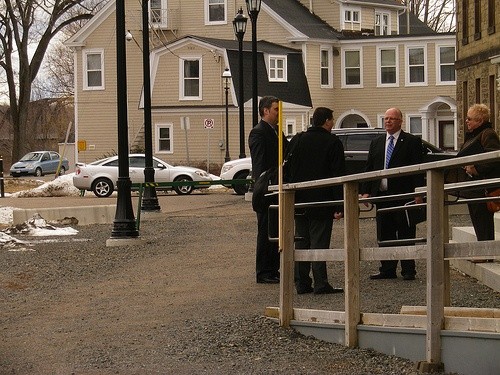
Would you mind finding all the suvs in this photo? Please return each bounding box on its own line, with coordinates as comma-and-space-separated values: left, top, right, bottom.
330, 128, 459, 196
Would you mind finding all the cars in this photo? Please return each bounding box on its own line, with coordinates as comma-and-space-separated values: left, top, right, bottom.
72, 154, 212, 198
10, 151, 69, 178
220, 134, 295, 195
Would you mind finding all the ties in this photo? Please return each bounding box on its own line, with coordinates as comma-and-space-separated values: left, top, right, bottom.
386, 136, 395, 168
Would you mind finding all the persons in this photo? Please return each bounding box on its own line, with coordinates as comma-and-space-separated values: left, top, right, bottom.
362, 108, 423, 280
248, 97, 290, 283
288, 107, 344, 295
455, 104, 500, 262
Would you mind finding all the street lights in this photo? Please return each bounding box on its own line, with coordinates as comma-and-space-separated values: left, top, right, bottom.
245, 0, 262, 201
232, 5, 248, 160
221, 67, 232, 163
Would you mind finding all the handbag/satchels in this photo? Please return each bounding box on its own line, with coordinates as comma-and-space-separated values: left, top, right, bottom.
484, 188, 500, 212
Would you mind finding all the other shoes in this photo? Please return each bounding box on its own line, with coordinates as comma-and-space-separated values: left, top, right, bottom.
403, 273, 415, 280
297, 286, 313, 294
257, 274, 281, 284
322, 286, 343, 293
467, 259, 493, 263
369, 271, 397, 278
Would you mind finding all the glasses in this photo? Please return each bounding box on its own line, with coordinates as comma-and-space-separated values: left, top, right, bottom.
465, 116, 472, 121
